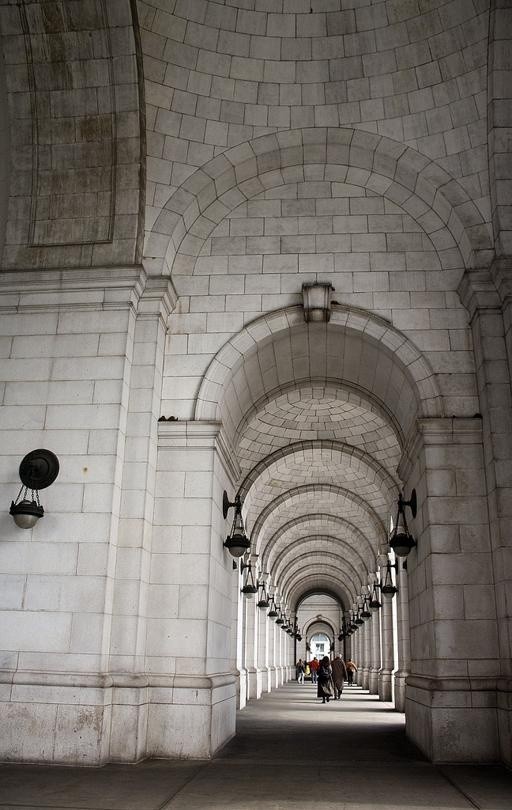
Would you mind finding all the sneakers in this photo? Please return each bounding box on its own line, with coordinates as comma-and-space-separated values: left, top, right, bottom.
298, 682, 305, 684
322, 697, 340, 703
348, 683, 353, 686
311, 681, 317, 684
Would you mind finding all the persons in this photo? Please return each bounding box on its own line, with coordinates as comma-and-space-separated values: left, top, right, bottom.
296, 656, 358, 703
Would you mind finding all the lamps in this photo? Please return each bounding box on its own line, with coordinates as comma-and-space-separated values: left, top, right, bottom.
298, 280, 334, 322
353, 489, 417, 626
9, 448, 59, 529
220, 489, 285, 627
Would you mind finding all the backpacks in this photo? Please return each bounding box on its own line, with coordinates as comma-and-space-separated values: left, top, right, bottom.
321, 666, 331, 681
